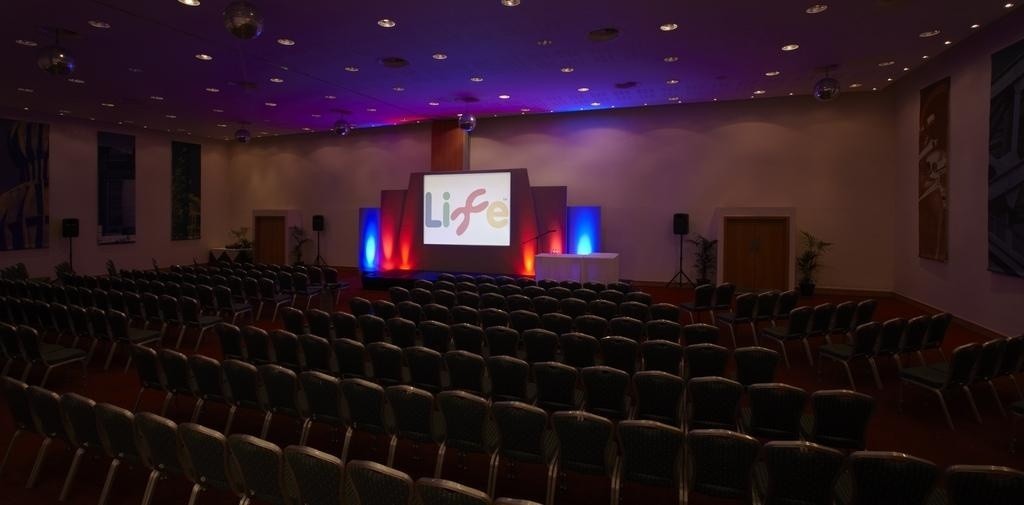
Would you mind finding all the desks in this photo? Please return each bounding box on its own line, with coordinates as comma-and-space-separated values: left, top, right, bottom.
535, 253, 620, 284
210, 248, 253, 264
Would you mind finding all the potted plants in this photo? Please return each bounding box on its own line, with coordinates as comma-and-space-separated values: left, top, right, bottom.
688, 232, 718, 286
795, 232, 833, 295
289, 225, 306, 266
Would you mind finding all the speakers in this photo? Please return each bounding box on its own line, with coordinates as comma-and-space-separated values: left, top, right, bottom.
312, 214, 324, 232
62, 218, 79, 238
672, 212, 690, 235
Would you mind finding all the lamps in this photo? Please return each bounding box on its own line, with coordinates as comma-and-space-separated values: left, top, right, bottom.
234, 121, 252, 143
812, 65, 841, 102
454, 96, 480, 133
333, 109, 351, 137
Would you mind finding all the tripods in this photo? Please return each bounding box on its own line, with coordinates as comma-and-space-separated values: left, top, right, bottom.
314, 236, 327, 266
664, 241, 695, 288
